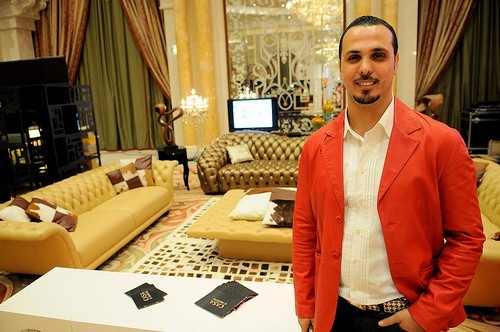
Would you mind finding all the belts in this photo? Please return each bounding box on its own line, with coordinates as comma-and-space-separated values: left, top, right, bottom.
346, 297, 410, 314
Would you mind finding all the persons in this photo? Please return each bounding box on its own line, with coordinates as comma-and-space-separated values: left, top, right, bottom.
291, 16, 486, 332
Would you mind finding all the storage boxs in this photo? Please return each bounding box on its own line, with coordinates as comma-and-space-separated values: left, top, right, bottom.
460, 111, 500, 154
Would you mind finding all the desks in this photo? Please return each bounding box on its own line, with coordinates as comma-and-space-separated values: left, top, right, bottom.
0, 266, 302, 332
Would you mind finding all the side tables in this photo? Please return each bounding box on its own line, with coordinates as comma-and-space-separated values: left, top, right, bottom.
158, 146, 190, 191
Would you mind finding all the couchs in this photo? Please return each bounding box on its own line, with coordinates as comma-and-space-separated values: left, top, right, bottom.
0, 159, 178, 275
197, 132, 309, 194
462, 154, 500, 307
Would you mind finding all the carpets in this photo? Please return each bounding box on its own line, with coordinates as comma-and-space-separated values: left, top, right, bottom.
129, 197, 292, 284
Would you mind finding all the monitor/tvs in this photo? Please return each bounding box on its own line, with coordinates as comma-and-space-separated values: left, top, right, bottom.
227, 98, 278, 132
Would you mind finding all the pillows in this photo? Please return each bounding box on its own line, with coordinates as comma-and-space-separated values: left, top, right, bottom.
105, 162, 143, 194
25, 198, 78, 232
225, 144, 253, 163
119, 154, 156, 186
0, 195, 36, 222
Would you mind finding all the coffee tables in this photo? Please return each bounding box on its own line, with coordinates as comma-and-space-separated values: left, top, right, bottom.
188, 189, 292, 263
